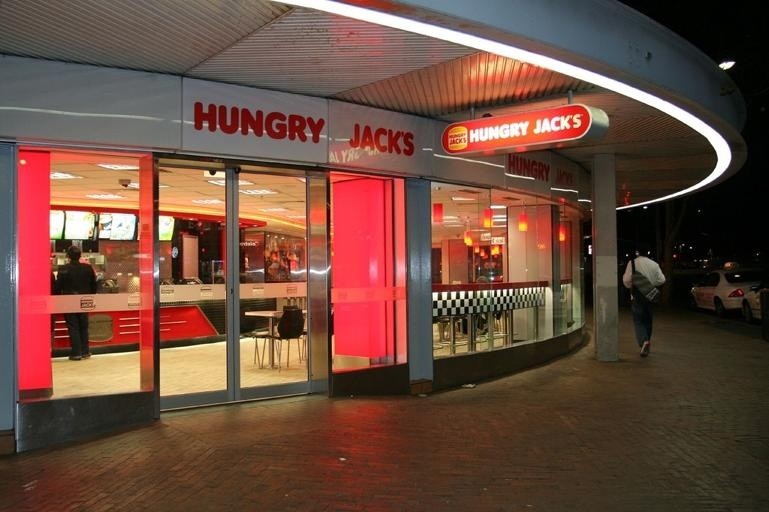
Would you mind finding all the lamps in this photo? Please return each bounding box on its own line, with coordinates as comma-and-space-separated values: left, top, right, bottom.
433, 187, 566, 261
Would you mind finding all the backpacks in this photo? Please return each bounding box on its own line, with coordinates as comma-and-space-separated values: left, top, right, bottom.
631, 259, 661, 308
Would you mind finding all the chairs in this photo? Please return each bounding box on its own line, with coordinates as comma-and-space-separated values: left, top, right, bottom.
433, 311, 516, 356
252, 302, 334, 373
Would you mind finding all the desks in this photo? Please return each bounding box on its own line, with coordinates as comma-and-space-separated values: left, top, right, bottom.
245, 310, 307, 370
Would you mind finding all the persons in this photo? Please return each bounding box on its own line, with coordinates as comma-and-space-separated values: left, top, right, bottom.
264, 251, 290, 283
620, 241, 667, 360
56, 245, 99, 360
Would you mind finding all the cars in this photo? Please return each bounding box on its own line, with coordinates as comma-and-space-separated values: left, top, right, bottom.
689, 262, 769, 322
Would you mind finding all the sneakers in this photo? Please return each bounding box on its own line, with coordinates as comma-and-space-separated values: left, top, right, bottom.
69, 350, 92, 361
639, 340, 652, 357
